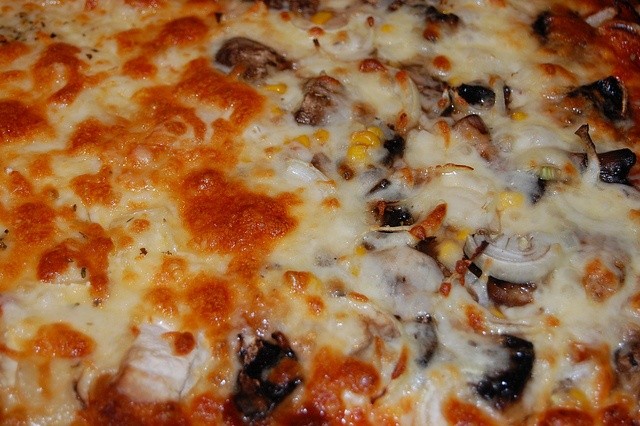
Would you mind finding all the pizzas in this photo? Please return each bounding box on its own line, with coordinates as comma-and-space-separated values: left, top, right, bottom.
0, 0, 636, 426
